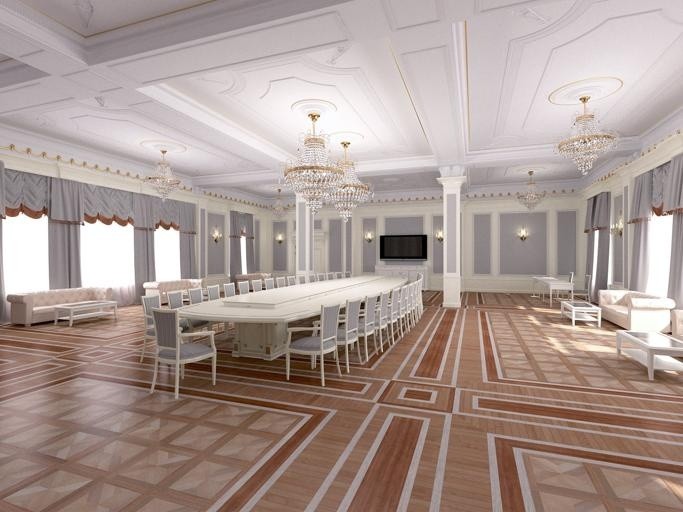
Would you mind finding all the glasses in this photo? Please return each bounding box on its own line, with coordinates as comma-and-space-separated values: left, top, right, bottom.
283, 99, 346, 216
364, 229, 375, 243
211, 224, 223, 244
144, 150, 181, 202
436, 229, 443, 243
515, 171, 549, 210
615, 215, 624, 237
329, 132, 374, 223
516, 225, 528, 241
275, 232, 284, 244
557, 96, 618, 176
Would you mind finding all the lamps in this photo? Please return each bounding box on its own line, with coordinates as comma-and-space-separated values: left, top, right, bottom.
670, 309, 683, 342
556, 272, 574, 302
568, 275, 591, 303
150, 306, 217, 400
140, 271, 423, 386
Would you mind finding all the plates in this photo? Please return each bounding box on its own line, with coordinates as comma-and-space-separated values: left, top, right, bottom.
375, 265, 427, 292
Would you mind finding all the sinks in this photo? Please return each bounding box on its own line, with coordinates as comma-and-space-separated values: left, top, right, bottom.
598, 290, 676, 329
7, 288, 113, 327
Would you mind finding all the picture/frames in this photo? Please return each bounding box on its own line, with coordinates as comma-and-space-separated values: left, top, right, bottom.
528, 275, 573, 308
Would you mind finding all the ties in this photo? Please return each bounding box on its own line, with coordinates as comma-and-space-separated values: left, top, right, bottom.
380, 235, 428, 260
